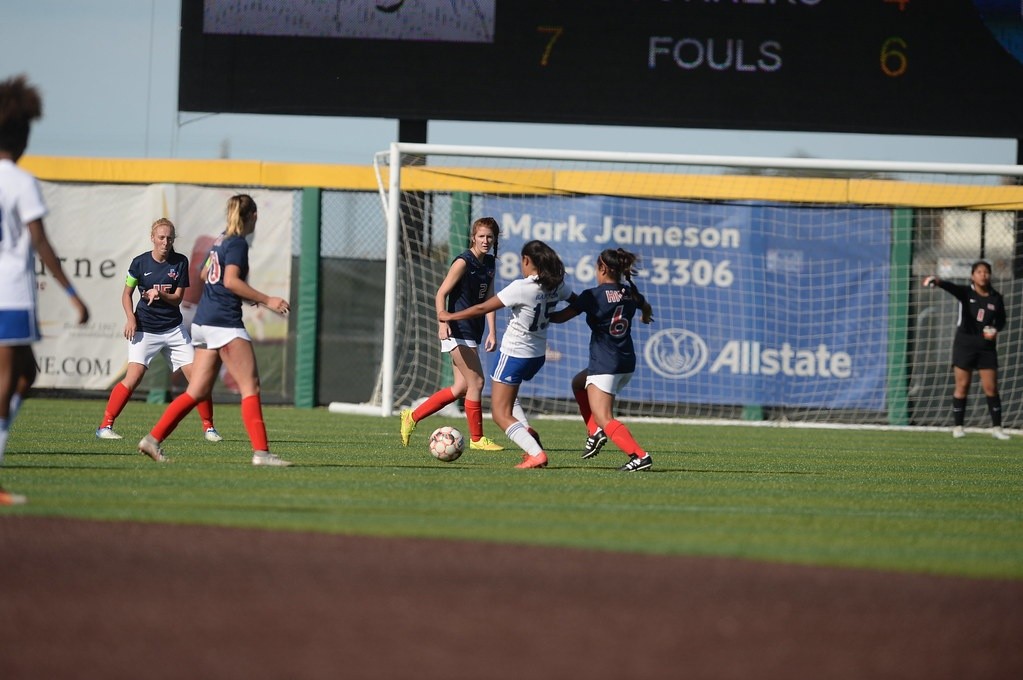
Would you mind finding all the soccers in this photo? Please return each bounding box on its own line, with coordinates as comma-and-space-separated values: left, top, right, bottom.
429, 426, 465, 462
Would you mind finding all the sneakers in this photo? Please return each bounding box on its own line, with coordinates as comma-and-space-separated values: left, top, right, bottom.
205, 427, 223, 442
526, 426, 544, 449
138, 435, 170, 463
515, 450, 548, 468
620, 451, 652, 473
470, 435, 505, 450
581, 429, 608, 459
399, 409, 418, 447
252, 452, 294, 467
95, 426, 124, 440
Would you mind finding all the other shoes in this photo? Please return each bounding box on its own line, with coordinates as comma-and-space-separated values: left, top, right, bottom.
952, 425, 965, 439
990, 427, 1011, 440
0, 485, 26, 504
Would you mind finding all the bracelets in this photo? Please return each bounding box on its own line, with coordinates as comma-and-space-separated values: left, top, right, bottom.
66, 286, 75, 297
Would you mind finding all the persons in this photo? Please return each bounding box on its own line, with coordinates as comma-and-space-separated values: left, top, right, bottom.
400, 217, 504, 451
0, 72, 90, 504
550, 248, 655, 472
922, 261, 1010, 440
137, 194, 292, 467
95, 218, 223, 442
438, 241, 579, 469
